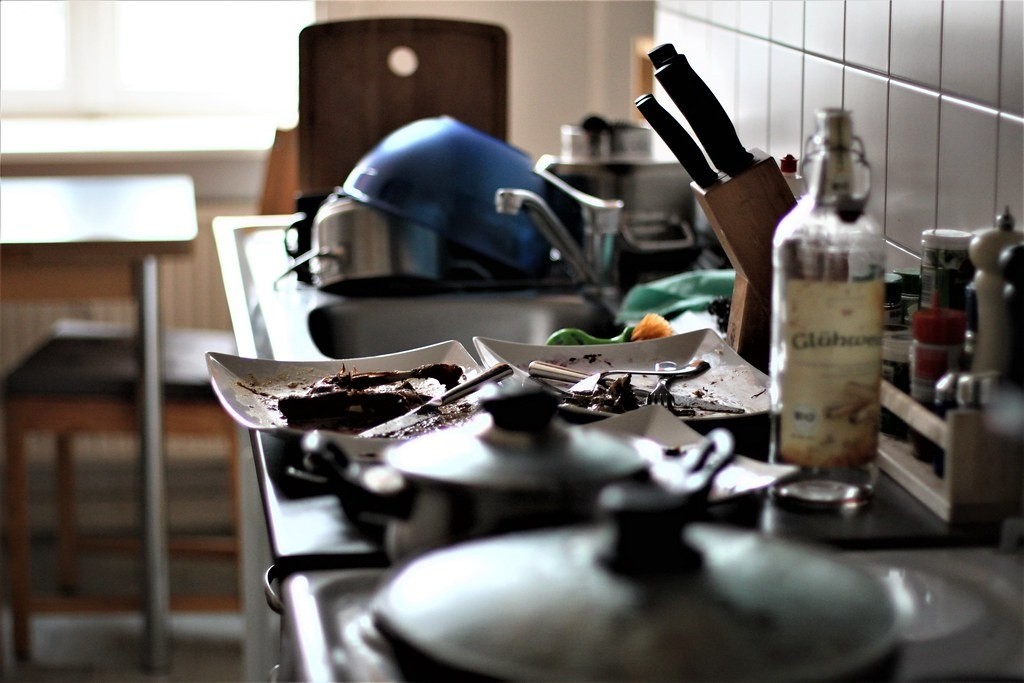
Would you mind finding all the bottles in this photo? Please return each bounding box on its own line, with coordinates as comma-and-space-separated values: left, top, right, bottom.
882, 268, 922, 323
770, 108, 886, 509
775, 155, 803, 204
910, 310, 968, 469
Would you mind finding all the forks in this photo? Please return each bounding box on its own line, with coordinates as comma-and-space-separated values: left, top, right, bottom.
647, 361, 677, 411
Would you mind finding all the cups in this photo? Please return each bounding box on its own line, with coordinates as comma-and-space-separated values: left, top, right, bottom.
284, 193, 328, 284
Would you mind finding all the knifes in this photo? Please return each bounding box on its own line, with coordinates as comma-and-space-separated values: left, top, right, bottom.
355, 363, 514, 437
528, 360, 745, 413
634, 42, 753, 184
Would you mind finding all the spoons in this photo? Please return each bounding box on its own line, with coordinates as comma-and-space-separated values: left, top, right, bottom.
569, 359, 711, 391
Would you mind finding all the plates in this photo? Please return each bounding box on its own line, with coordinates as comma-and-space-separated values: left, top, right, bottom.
472, 328, 770, 420
205, 340, 502, 440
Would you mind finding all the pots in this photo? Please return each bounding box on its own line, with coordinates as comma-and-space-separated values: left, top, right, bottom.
301, 385, 736, 568
370, 484, 901, 683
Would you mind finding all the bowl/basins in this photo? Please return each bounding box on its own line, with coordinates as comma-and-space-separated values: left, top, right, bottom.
310, 114, 551, 293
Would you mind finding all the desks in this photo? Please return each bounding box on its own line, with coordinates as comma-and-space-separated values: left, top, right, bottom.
0, 171, 200, 673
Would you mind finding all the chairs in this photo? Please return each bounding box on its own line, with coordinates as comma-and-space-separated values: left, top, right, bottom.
0, 117, 301, 664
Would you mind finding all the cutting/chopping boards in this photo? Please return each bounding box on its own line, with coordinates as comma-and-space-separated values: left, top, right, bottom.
297, 17, 509, 187
